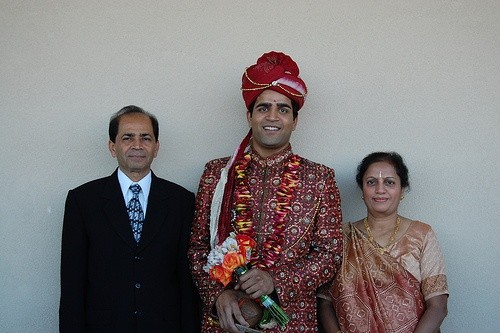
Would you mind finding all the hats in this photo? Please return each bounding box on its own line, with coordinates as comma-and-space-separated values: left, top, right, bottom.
242, 51, 307, 110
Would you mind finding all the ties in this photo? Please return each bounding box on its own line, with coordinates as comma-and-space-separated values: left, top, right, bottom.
127, 184, 145, 246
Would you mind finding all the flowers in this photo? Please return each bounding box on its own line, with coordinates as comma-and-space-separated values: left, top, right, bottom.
203, 230, 292, 330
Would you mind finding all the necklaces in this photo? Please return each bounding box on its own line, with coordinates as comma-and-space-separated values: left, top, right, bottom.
362, 215, 402, 255
232, 150, 302, 267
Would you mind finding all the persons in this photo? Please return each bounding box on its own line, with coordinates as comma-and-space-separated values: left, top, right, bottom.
58, 104, 206, 332
187, 50, 343, 333
317, 152, 449, 333
